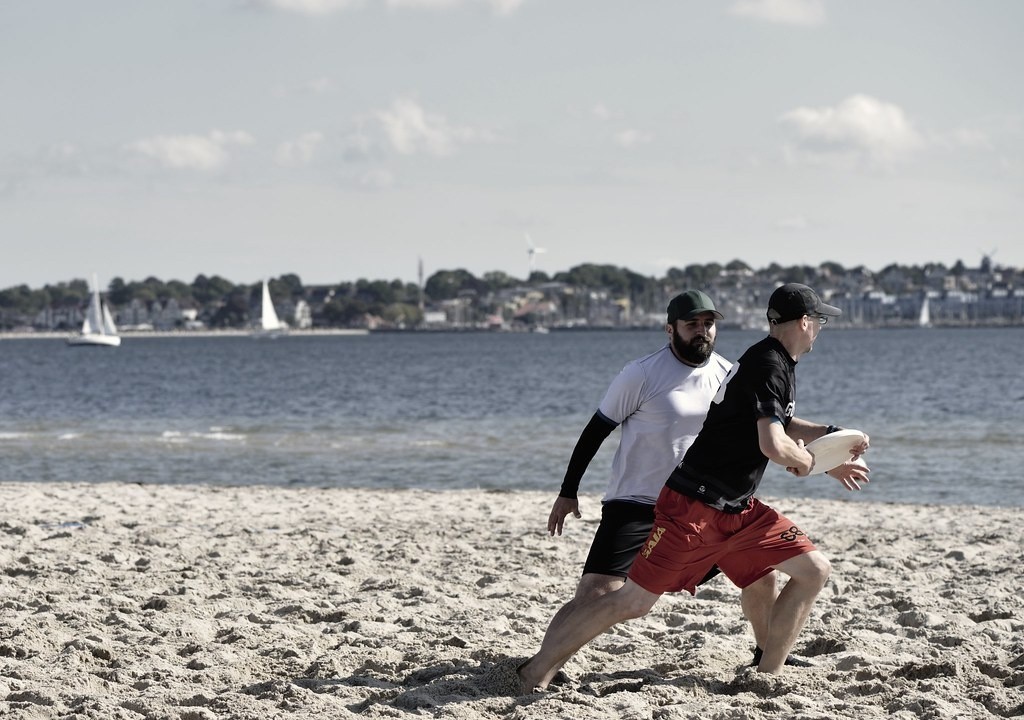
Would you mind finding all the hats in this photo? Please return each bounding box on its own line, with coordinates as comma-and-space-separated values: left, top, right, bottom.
767, 283, 842, 325
667, 290, 724, 320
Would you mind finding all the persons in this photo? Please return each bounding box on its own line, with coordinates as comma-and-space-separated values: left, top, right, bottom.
516, 283, 869, 695
543, 291, 871, 670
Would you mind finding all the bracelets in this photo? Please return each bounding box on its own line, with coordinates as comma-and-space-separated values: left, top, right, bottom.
827, 425, 840, 436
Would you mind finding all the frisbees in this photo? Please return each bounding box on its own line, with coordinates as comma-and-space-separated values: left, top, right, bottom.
805, 429, 865, 476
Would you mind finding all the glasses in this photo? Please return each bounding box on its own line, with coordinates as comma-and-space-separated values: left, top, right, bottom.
810, 315, 828, 324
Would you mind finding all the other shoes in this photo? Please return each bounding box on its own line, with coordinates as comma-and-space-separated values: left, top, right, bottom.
517, 661, 576, 685
751, 646, 815, 668
